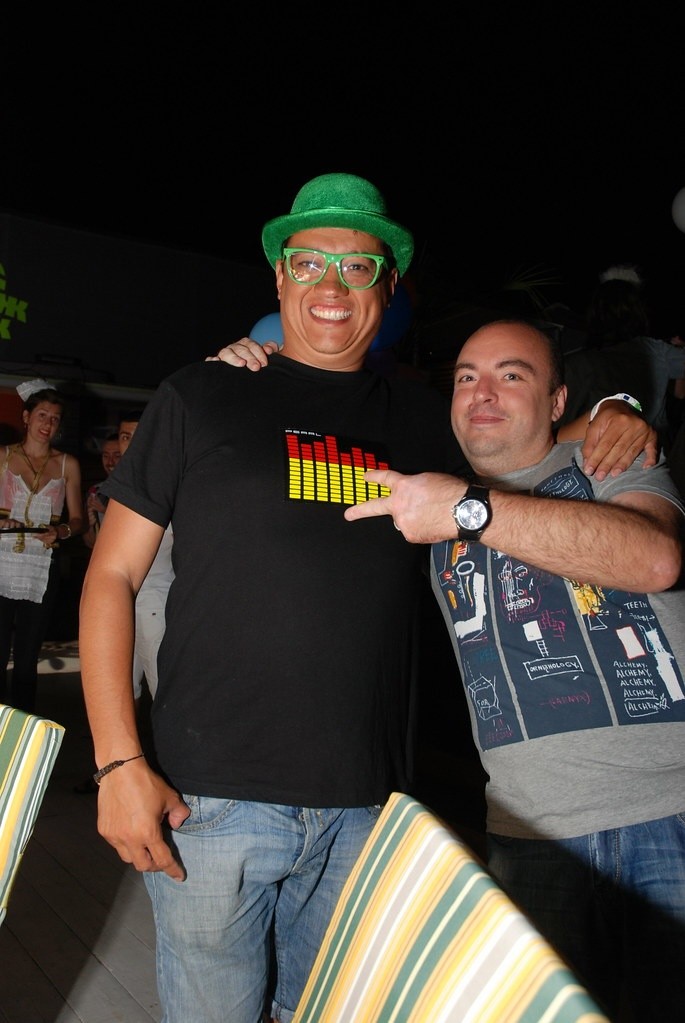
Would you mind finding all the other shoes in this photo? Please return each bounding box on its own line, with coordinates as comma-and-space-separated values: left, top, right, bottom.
74, 778, 99, 793
80, 722, 92, 737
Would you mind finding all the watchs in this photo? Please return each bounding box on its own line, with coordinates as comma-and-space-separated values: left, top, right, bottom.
452, 483, 492, 541
590, 393, 642, 422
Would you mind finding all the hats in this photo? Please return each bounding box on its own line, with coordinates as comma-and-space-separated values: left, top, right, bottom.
262, 173, 414, 279
16, 379, 56, 403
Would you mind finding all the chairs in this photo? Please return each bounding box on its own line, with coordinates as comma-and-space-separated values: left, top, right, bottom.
0, 703, 65, 926
288, 791, 617, 1023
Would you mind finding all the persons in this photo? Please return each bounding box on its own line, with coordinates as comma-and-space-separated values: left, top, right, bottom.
204, 318, 685, 1023
568, 280, 684, 458
79, 174, 684, 1022
0, 379, 145, 713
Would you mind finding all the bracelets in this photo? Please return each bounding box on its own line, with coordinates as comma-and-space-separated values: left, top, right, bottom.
93, 754, 144, 785
60, 524, 72, 539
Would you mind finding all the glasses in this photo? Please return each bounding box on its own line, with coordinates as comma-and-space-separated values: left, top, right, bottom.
282, 248, 389, 290
